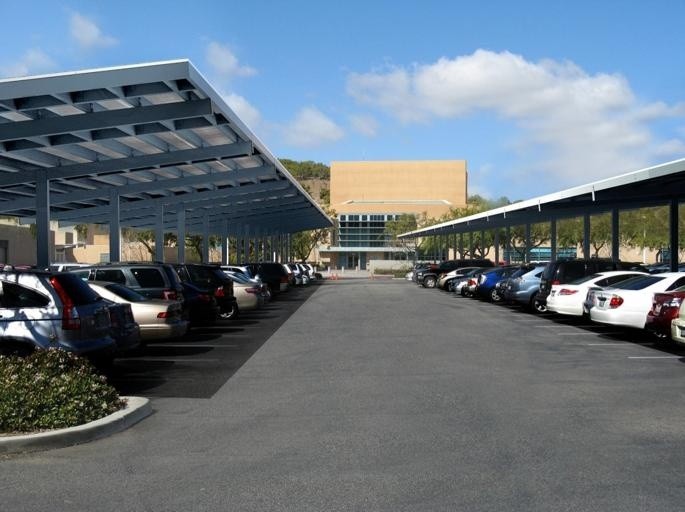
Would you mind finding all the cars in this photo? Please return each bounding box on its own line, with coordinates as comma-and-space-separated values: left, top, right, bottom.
410, 256, 685, 346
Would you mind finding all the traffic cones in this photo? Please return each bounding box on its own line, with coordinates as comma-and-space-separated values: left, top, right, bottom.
331, 273, 334, 281
335, 273, 339, 280
